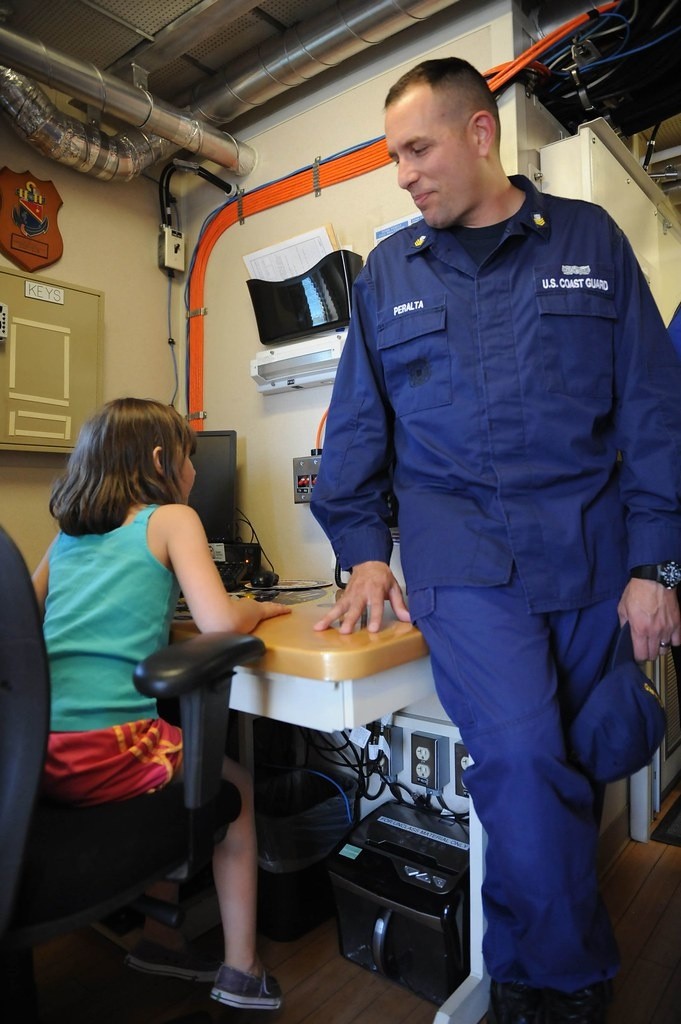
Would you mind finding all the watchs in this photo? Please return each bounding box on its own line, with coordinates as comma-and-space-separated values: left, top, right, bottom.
630, 560, 681, 590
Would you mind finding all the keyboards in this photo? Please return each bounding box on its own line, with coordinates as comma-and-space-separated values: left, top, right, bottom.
215, 560, 248, 592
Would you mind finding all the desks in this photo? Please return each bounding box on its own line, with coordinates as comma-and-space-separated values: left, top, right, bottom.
174, 577, 436, 734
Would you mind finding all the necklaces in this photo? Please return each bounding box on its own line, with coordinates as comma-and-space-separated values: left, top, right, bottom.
660, 641, 672, 648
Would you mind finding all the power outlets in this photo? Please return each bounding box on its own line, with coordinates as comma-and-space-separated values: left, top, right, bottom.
411, 731, 450, 790
454, 740, 470, 798
365, 725, 403, 776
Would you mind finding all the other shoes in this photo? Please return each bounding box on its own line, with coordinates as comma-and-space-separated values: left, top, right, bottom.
478, 982, 612, 1024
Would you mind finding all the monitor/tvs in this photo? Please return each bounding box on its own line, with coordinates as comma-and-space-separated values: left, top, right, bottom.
185, 430, 236, 543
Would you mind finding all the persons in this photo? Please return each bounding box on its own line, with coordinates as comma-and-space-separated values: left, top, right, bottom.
31, 398, 291, 1009
309, 56, 681, 1024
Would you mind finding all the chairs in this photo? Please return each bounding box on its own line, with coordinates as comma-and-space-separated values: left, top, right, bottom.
0, 520, 267, 1024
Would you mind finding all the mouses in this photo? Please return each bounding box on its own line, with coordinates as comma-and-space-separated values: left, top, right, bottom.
251, 571, 279, 589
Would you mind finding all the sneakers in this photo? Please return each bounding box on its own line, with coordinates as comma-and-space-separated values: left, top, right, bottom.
210, 963, 283, 1010
124, 938, 218, 982
